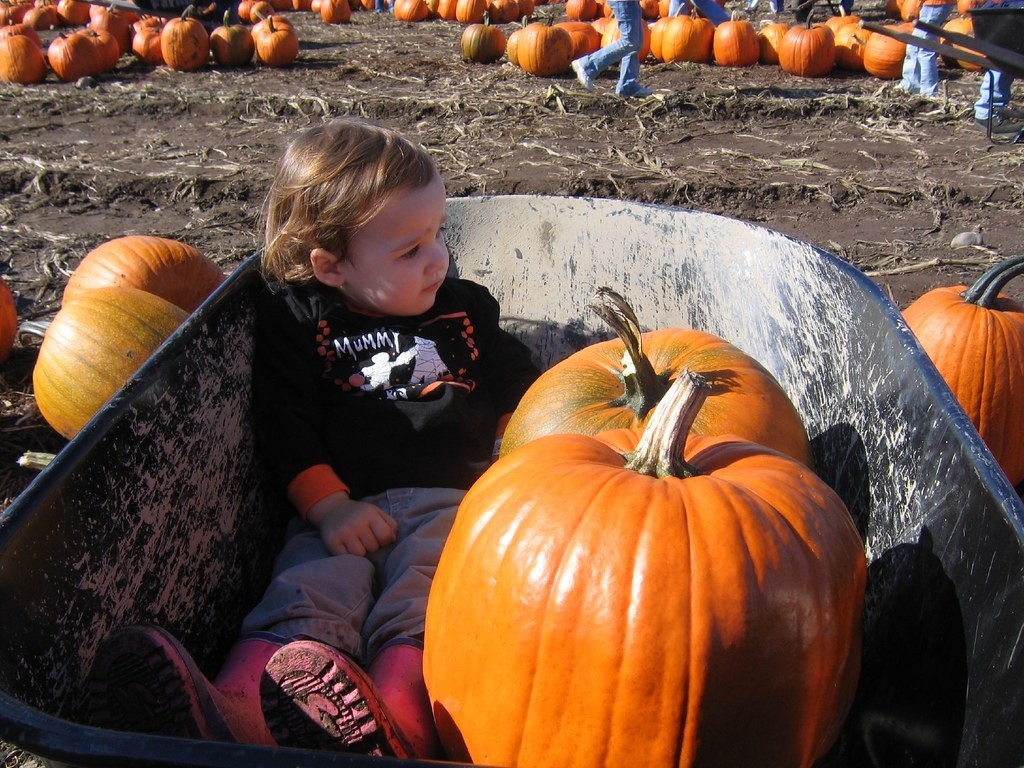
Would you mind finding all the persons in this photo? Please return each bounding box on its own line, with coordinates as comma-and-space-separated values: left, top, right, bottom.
97, 121, 541, 758
669, 0, 730, 27
895, 0, 959, 100
373, 0, 395, 14
747, 0, 785, 17
838, 0, 854, 17
972, 0, 1024, 139
181, 0, 241, 25
572, 0, 654, 98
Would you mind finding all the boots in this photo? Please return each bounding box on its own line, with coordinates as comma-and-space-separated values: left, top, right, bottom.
88, 623, 284, 749
260, 638, 443, 760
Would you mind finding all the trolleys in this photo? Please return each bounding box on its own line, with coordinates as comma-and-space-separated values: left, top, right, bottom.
0, 191, 1024, 768
858, 7, 1024, 146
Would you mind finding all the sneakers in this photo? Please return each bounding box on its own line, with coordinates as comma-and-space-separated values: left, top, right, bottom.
633, 88, 652, 97
995, 106, 1024, 120
976, 112, 1023, 138
571, 60, 594, 92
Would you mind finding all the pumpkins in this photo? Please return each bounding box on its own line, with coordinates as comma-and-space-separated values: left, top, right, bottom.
899, 254, 1024, 491
0, 0, 998, 86
423, 367, 869, 768
0, 234, 233, 439
499, 288, 812, 472
